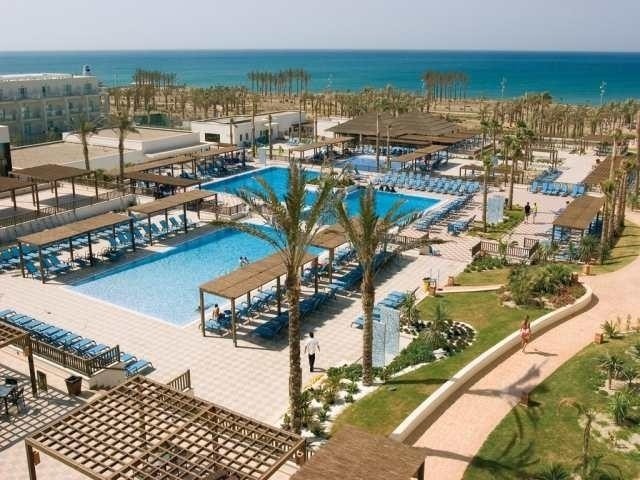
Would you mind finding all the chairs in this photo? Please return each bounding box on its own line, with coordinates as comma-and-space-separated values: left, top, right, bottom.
311, 142, 479, 235
526, 178, 604, 243
1, 147, 246, 282
0, 308, 155, 417
202, 246, 410, 346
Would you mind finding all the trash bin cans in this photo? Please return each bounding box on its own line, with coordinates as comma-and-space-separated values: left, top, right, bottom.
65, 376, 83, 396
571, 272, 578, 282
595, 334, 603, 344
448, 276, 454, 286
583, 265, 590, 275
